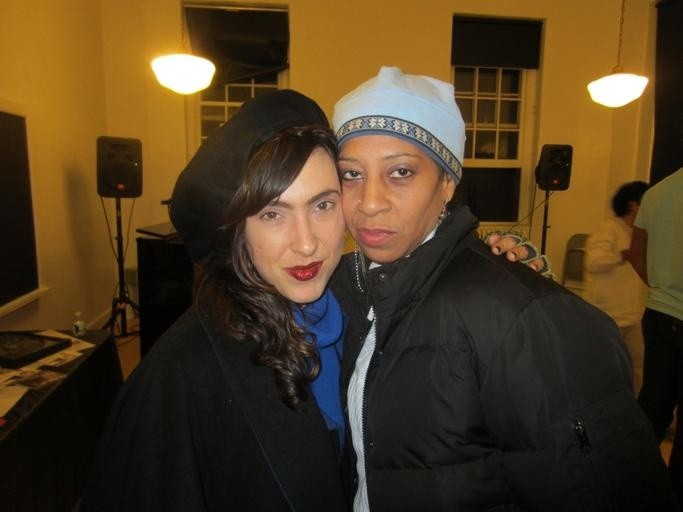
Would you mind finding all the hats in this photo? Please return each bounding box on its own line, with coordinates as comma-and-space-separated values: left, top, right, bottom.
169, 90, 330, 263
332, 66, 467, 182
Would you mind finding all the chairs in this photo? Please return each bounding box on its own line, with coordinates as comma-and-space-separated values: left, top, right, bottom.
558, 233, 590, 291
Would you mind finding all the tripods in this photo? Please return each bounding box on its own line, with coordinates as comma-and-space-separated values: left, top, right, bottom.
101, 198, 140, 336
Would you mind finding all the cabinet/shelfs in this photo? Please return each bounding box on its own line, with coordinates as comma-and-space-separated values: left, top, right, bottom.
0, 327, 127, 511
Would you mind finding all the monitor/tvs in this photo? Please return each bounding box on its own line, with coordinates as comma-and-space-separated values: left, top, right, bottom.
1, 111, 40, 307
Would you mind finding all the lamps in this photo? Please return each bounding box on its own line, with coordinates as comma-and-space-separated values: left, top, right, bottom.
149, 1, 217, 97
585, 1, 651, 112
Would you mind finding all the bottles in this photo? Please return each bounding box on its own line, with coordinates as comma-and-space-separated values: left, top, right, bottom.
72, 311, 87, 338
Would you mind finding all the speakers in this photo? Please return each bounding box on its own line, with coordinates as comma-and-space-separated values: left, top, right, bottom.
97, 136, 143, 198
534, 144, 572, 190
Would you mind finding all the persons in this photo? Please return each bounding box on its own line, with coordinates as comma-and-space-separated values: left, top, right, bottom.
95, 86, 558, 512
626, 160, 682, 490
578, 182, 646, 402
329, 65, 682, 512
